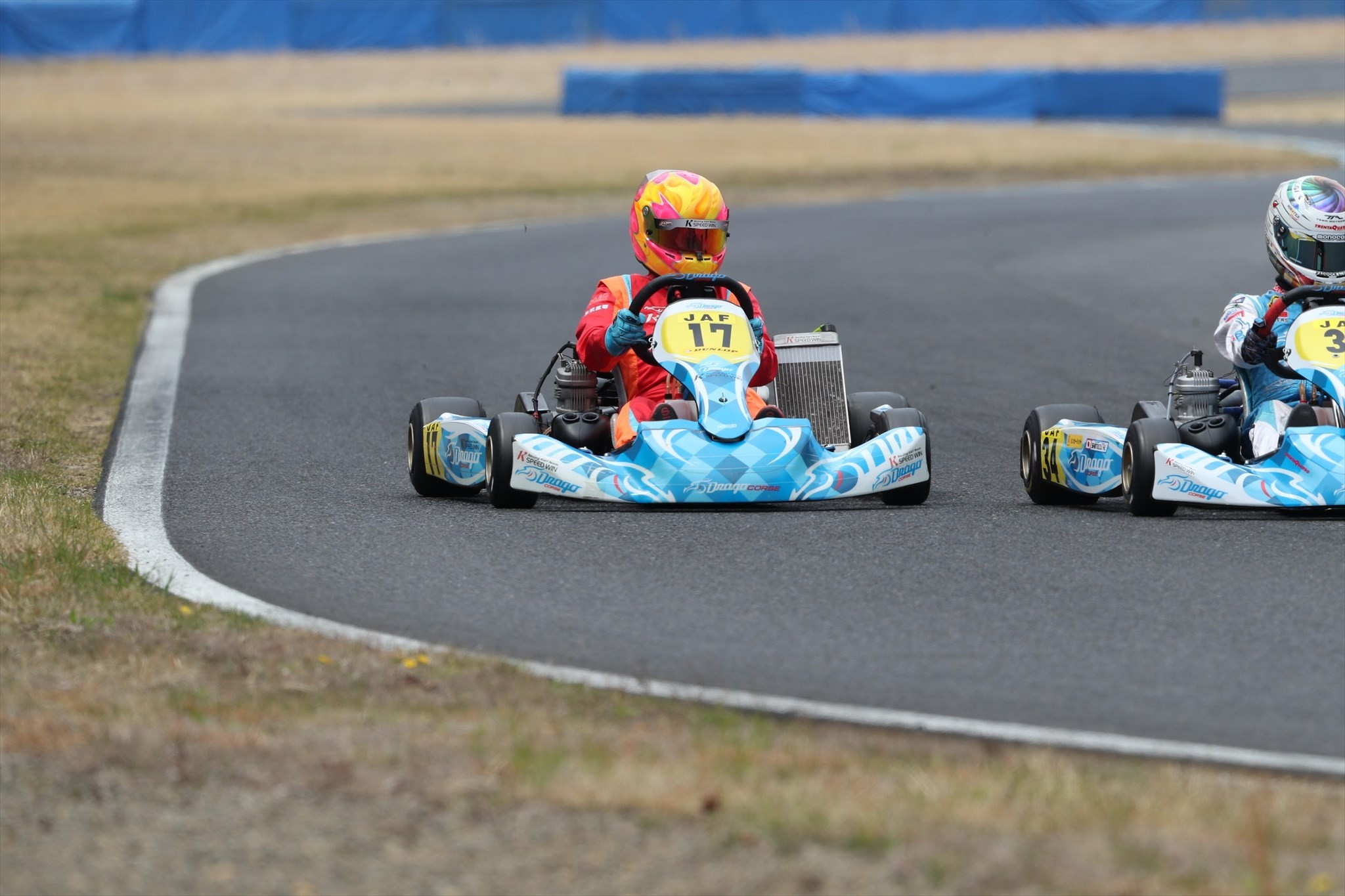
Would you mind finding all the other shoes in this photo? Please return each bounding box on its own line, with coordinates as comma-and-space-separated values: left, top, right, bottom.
1283, 402, 1318, 431
649, 403, 679, 421
754, 404, 785, 420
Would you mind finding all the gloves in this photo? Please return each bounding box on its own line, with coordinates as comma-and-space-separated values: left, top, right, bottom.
750, 317, 764, 354
1242, 318, 1277, 365
604, 308, 648, 356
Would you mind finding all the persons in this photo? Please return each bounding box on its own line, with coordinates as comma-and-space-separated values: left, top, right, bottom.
1214, 176, 1345, 465
575, 170, 785, 452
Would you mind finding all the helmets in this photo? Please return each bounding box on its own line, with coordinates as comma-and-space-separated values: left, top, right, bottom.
629, 170, 728, 276
1264, 175, 1345, 291
